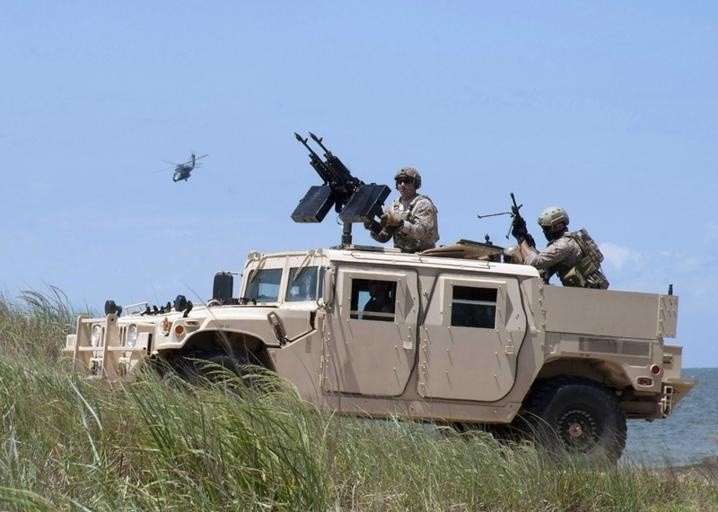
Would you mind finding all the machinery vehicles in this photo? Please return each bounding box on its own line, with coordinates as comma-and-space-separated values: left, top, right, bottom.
50, 128, 700, 473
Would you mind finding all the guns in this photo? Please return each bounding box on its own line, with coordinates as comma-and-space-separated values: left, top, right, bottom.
295, 132, 396, 242
477, 192, 549, 284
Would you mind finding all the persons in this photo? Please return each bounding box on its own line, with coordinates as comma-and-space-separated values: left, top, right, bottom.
365, 167, 439, 254
510, 206, 608, 289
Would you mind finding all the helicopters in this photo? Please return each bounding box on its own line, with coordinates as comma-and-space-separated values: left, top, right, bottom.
150, 151, 209, 185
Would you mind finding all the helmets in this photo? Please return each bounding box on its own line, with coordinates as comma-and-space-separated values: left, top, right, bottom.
536, 206, 570, 227
503, 246, 522, 263
394, 167, 422, 188
